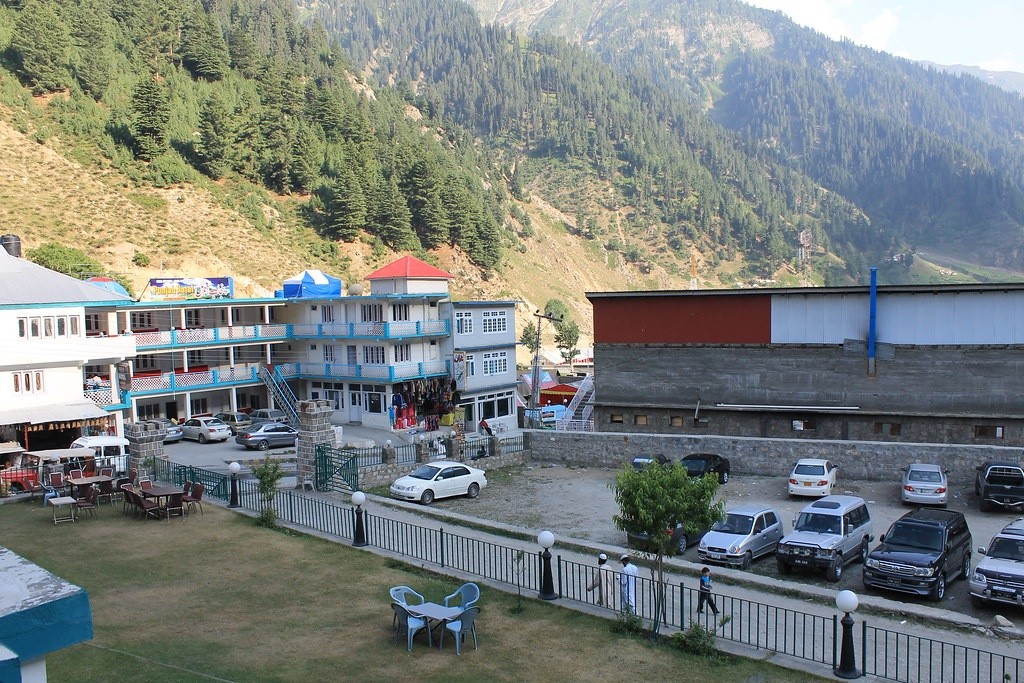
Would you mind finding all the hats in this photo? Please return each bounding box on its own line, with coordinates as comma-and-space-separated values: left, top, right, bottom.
621, 555, 629, 561
599, 554, 607, 559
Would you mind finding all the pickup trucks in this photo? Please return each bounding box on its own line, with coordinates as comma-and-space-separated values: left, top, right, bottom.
974, 459, 1024, 514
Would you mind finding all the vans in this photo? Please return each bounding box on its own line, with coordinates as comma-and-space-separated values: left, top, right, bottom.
70, 435, 130, 478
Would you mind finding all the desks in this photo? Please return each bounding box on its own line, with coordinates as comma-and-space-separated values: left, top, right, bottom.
409, 602, 458, 646
48, 496, 76, 524
68, 475, 111, 504
139, 487, 185, 518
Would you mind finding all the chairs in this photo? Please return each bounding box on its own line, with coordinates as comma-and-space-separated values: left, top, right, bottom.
114, 468, 204, 518
24, 468, 113, 504
390, 585, 432, 651
440, 583, 481, 655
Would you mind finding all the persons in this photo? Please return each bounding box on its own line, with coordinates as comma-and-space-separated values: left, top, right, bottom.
478, 446, 485, 454
436, 437, 446, 454
696, 567, 720, 615
588, 554, 613, 609
480, 417, 493, 436
88, 374, 101, 382
617, 555, 638, 615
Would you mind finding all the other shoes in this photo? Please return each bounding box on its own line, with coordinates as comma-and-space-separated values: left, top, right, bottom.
714, 611, 719, 613
697, 609, 703, 613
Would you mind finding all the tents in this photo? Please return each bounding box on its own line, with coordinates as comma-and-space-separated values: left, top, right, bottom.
283, 270, 341, 298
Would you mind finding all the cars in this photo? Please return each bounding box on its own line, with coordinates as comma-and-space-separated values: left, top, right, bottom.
389, 460, 489, 506
899, 463, 951, 508
235, 423, 302, 451
626, 518, 720, 555
696, 505, 784, 570
207, 410, 252, 436
629, 453, 672, 474
178, 416, 233, 444
786, 458, 838, 499
136, 418, 183, 442
248, 408, 289, 425
673, 452, 731, 484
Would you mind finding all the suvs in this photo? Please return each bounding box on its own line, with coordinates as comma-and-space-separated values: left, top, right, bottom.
0, 448, 96, 496
774, 494, 874, 584
967, 516, 1024, 609
861, 506, 974, 603
